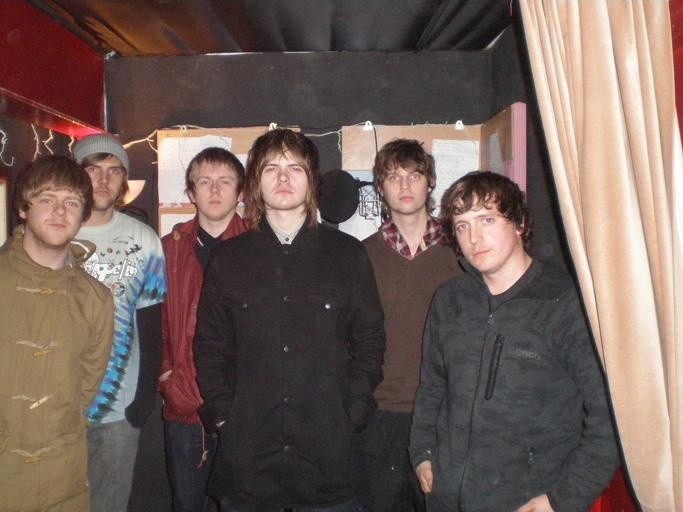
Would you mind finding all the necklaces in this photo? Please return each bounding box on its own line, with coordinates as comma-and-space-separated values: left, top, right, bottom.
270, 223, 304, 242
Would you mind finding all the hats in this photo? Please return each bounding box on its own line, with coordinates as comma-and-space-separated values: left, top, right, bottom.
73, 134, 130, 170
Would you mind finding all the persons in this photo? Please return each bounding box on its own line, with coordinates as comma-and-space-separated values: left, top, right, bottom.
357, 138, 465, 511
64, 133, 168, 510
192, 127, 385, 512
0, 154, 114, 511
158, 147, 256, 509
408, 169, 624, 512
